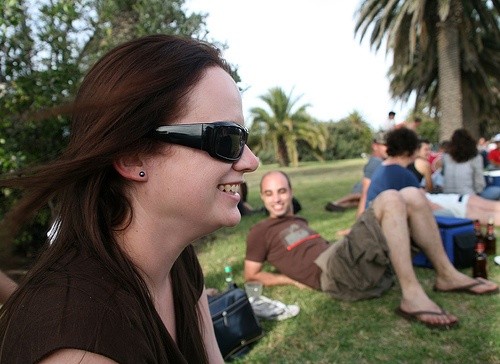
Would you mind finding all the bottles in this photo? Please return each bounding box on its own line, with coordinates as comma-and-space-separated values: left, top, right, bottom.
222, 266, 235, 294
473, 216, 496, 280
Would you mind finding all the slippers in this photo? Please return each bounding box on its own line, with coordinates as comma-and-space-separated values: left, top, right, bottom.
433, 279, 500, 295
394, 302, 458, 328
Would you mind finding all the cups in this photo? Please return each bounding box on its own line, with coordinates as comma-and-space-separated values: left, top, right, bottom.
244, 281, 263, 298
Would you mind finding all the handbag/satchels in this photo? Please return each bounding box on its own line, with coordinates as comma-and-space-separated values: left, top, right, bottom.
207, 284, 265, 359
411, 215, 477, 269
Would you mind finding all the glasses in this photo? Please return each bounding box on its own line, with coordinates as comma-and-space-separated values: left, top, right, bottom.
147, 121, 249, 164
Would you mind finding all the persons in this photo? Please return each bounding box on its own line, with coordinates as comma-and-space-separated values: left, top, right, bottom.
357, 132, 499, 227
379, 111, 398, 134
334, 182, 363, 207
405, 140, 444, 194
0, 34, 258, 364
439, 129, 500, 201
365, 129, 455, 218
243, 170, 500, 329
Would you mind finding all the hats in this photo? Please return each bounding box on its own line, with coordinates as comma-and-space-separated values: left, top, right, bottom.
373, 131, 387, 144
